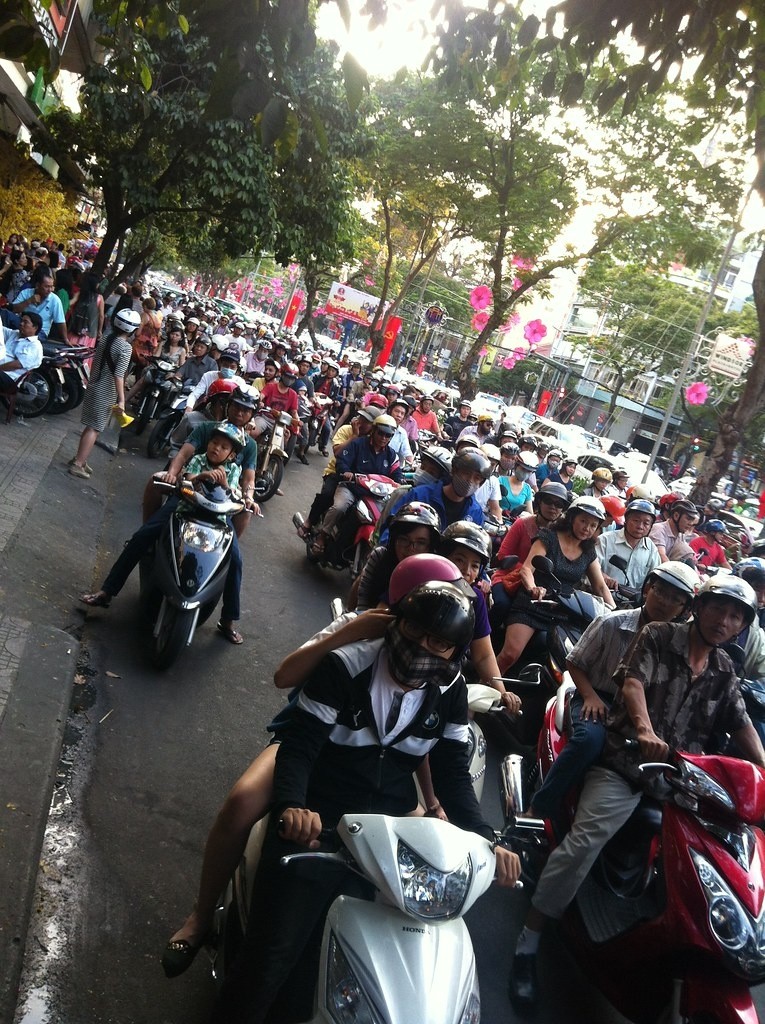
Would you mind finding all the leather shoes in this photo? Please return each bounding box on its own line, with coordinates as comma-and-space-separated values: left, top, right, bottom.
507, 952, 539, 1015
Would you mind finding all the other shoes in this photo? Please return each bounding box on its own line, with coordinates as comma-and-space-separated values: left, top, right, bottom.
160, 924, 216, 979
68, 455, 93, 480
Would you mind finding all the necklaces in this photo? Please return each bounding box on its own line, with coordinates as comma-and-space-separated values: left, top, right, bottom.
691, 654, 709, 679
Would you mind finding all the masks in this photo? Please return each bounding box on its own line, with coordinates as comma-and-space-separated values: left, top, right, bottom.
515, 467, 533, 482
451, 472, 480, 498
501, 459, 516, 470
282, 376, 295, 387
258, 350, 268, 361
412, 467, 440, 487
388, 627, 461, 689
220, 367, 236, 378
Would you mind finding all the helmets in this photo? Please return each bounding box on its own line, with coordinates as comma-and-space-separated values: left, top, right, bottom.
650, 560, 701, 600
438, 521, 494, 561
30, 241, 763, 600
390, 579, 476, 656
195, 334, 212, 353
387, 501, 442, 530
697, 574, 758, 624
387, 552, 478, 607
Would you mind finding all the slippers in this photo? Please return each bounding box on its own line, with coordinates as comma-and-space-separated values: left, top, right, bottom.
217, 621, 243, 644
78, 595, 110, 610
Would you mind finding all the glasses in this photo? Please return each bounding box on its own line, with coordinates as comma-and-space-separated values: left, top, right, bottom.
651, 583, 687, 607
541, 497, 565, 510
377, 430, 393, 438
399, 614, 458, 653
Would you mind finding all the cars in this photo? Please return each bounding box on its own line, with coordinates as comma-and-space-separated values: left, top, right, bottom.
157, 282, 189, 303
469, 391, 765, 557
213, 296, 461, 408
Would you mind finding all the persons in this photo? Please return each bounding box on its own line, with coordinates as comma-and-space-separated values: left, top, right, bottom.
0, 233, 765, 1009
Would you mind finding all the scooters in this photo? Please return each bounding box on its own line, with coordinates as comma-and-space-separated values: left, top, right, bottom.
0, 338, 99, 424
138, 473, 265, 673
130, 352, 343, 503
288, 407, 765, 749
201, 817, 548, 1024
499, 672, 765, 1024
